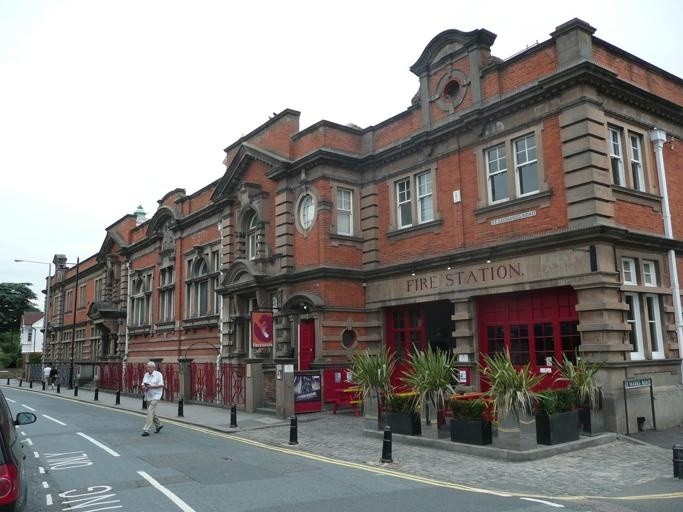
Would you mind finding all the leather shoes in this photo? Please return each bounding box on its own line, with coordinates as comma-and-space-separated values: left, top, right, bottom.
142, 432, 148, 436
154, 426, 162, 433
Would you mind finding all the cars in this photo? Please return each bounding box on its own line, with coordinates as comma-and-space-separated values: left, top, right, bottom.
0, 391, 37, 511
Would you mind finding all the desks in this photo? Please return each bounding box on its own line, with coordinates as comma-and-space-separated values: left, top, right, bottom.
332, 389, 363, 417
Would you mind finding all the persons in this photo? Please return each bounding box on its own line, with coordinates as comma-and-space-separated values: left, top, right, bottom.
139, 361, 164, 437
42, 364, 51, 391
49, 365, 57, 390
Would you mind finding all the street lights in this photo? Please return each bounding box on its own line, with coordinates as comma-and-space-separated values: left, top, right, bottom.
21, 326, 36, 352
15, 259, 50, 365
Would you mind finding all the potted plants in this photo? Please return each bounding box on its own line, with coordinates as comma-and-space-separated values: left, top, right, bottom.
341, 343, 610, 452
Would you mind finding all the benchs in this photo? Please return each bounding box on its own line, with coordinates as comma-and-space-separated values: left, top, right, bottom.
327, 398, 363, 417
